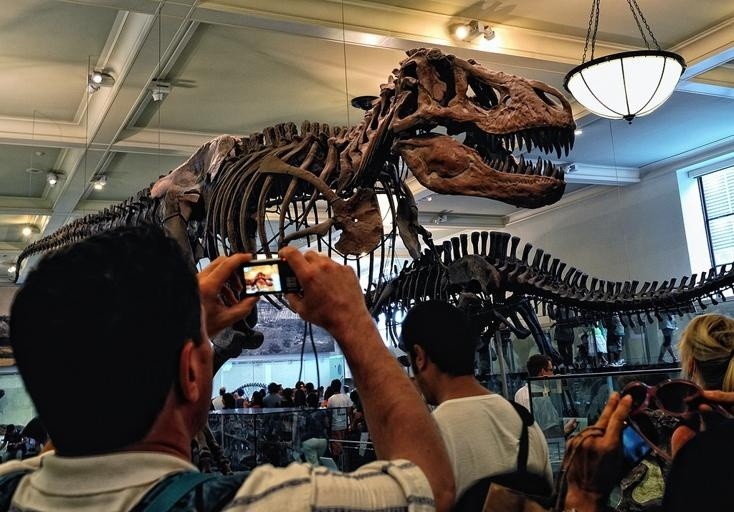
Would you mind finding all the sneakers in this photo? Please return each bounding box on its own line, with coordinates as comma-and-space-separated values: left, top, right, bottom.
609, 361, 623, 367
603, 361, 615, 368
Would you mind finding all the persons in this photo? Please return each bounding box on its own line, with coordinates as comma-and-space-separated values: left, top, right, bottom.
191, 379, 377, 475
0, 225, 456, 512
514, 312, 734, 512
0, 424, 27, 460
401, 301, 554, 512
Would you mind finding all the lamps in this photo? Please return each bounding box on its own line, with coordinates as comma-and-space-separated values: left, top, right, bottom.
86, 71, 116, 94
562, 1, 686, 126
149, 78, 170, 102
455, 19, 495, 44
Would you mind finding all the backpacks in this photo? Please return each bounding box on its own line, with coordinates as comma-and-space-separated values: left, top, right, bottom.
454, 471, 552, 512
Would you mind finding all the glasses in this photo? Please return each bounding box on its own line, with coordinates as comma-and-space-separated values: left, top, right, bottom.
620, 380, 734, 464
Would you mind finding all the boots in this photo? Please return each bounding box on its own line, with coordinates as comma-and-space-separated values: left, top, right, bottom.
668, 345, 681, 363
658, 345, 669, 365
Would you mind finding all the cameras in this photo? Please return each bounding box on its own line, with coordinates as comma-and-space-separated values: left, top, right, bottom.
238, 258, 304, 297
621, 411, 663, 473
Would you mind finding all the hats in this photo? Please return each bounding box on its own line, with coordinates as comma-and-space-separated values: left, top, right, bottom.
268, 383, 281, 393
